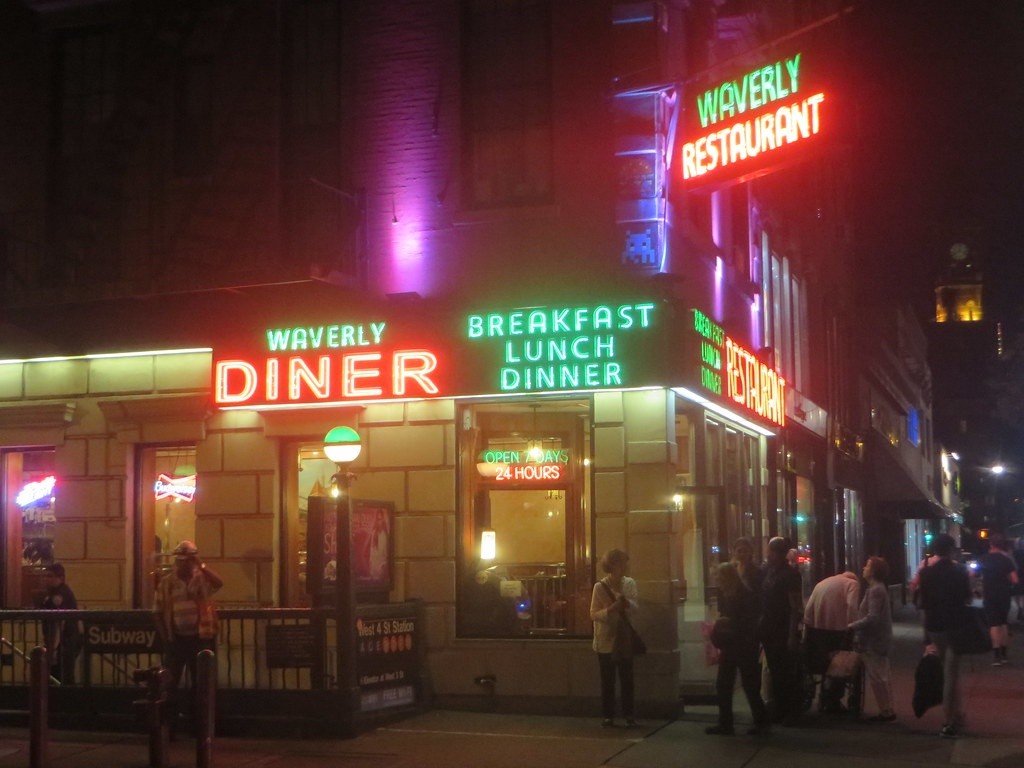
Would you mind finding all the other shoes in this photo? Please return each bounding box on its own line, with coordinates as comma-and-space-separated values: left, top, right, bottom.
600, 718, 613, 728
625, 720, 637, 728
867, 715, 898, 724
747, 724, 761, 735
706, 725, 735, 736
940, 729, 967, 738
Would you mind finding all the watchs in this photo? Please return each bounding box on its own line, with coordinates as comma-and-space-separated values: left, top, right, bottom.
201, 564, 206, 568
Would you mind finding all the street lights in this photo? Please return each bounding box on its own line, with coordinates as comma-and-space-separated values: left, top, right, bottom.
321, 425, 361, 738
991, 461, 1004, 538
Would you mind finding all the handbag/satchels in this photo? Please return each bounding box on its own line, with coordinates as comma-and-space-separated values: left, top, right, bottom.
700, 621, 722, 665
631, 633, 646, 654
949, 607, 992, 654
825, 651, 860, 680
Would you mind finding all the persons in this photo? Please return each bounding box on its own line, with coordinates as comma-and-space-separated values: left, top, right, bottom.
847, 557, 898, 723
969, 533, 1019, 666
590, 548, 640, 730
702, 537, 771, 735
39, 563, 82, 684
802, 571, 861, 715
919, 534, 975, 738
757, 537, 802, 726
1010, 536, 1024, 619
913, 539, 941, 646
152, 541, 223, 739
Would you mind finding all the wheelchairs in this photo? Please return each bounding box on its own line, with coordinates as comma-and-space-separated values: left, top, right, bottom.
794, 634, 864, 718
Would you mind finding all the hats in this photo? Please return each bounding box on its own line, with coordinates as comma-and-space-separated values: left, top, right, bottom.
173, 541, 198, 559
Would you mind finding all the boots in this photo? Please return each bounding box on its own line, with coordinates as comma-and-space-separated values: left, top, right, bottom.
992, 648, 1001, 666
1001, 646, 1008, 663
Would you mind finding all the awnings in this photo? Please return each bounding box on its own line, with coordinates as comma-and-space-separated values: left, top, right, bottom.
870, 437, 946, 519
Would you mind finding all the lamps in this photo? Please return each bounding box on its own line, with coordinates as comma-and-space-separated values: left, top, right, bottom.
759, 347, 772, 354
387, 291, 421, 307
653, 272, 682, 287
